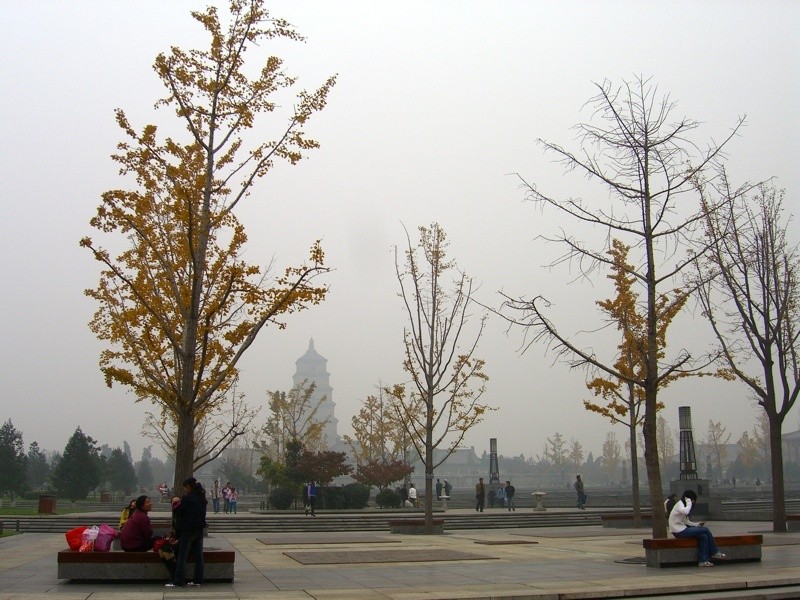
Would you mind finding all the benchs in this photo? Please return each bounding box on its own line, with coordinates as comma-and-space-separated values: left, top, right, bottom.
643, 535, 764, 568
601, 513, 669, 529
786, 512, 800, 532
153, 523, 209, 539
58, 547, 235, 584
389, 519, 444, 535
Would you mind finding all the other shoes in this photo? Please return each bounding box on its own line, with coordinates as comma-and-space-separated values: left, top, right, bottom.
697, 560, 714, 567
164, 582, 184, 587
187, 581, 201, 586
311, 514, 316, 517
712, 551, 726, 559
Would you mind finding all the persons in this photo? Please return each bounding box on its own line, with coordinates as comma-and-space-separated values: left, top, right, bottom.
120, 495, 164, 587
164, 478, 208, 588
663, 493, 679, 527
208, 480, 237, 515
436, 479, 452, 501
475, 478, 485, 512
158, 483, 174, 503
395, 483, 416, 508
118, 499, 137, 530
305, 480, 317, 517
487, 480, 516, 512
574, 475, 586, 510
668, 489, 727, 567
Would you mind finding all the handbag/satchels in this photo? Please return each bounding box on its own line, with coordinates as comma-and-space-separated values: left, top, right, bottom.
305, 505, 310, 511
65, 524, 119, 552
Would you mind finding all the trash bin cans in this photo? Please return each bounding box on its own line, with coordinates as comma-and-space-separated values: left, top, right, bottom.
101, 490, 112, 502
37, 495, 56, 513
